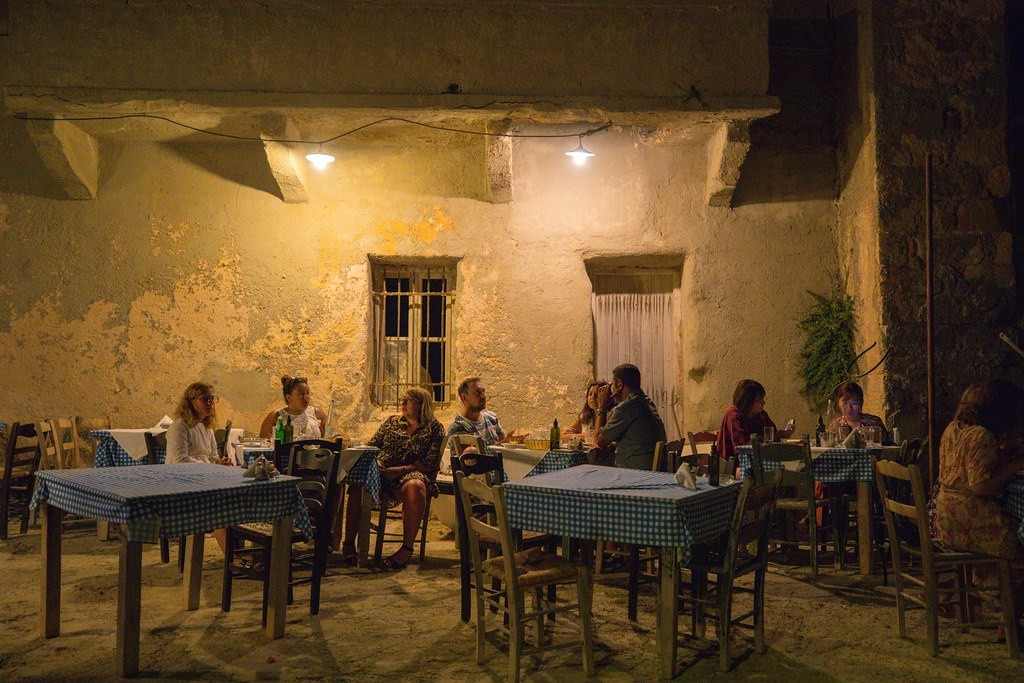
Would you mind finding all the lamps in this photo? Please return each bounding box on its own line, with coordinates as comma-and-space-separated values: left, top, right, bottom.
565, 134, 594, 166
306, 142, 335, 171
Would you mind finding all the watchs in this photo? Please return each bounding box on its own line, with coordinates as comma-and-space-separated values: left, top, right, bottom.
595, 408, 606, 416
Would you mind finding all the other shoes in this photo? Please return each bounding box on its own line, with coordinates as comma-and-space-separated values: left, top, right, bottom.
234, 558, 253, 569
917, 593, 956, 619
594, 551, 622, 572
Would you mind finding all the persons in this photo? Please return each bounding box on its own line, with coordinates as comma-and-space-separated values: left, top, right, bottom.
595, 363, 667, 571
717, 379, 796, 526
343, 387, 446, 571
930, 384, 1024, 645
560, 381, 617, 450
166, 382, 262, 576
448, 377, 529, 541
805, 381, 888, 525
259, 374, 336, 553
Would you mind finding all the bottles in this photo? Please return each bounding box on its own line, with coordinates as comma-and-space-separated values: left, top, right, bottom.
275, 416, 284, 444
825, 399, 839, 448
815, 415, 825, 447
549, 417, 560, 451
325, 400, 339, 442
569, 436, 583, 452
284, 415, 293, 444
708, 438, 719, 487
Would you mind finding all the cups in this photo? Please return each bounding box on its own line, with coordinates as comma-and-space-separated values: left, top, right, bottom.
764, 427, 774, 445
294, 426, 301, 442
585, 433, 594, 445
866, 426, 882, 449
839, 426, 852, 447
508, 426, 519, 445
564, 426, 573, 434
894, 427, 903, 446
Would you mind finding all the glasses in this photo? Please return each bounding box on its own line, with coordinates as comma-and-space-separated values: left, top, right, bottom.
289, 376, 296, 385
195, 397, 219, 404
399, 397, 420, 406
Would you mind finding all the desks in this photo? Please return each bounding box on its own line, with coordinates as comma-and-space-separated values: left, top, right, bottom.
502, 464, 743, 683
29, 462, 313, 678
230, 443, 380, 569
486, 446, 588, 481
737, 446, 904, 575
90, 429, 167, 468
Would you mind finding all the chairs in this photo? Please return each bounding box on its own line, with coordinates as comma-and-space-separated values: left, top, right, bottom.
0, 416, 1024, 683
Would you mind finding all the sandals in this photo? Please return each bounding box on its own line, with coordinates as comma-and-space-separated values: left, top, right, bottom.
342, 542, 357, 565
385, 544, 414, 569
